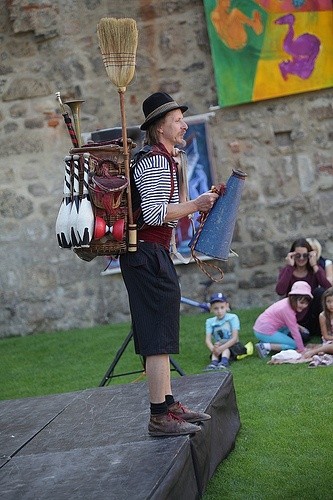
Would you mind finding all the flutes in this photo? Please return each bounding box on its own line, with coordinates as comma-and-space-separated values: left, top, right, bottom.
55, 92, 77, 149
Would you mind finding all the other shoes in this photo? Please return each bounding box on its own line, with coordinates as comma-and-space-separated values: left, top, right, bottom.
208, 360, 217, 369
217, 361, 229, 369
147, 409, 202, 436
167, 400, 212, 423
255, 343, 269, 360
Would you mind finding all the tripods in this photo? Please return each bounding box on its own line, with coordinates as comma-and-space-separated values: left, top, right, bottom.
98, 327, 185, 388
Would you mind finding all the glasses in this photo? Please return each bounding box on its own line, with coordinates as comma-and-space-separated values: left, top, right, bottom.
294, 253, 309, 259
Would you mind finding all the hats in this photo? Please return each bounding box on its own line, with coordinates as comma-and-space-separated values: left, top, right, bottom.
288, 281, 313, 299
210, 292, 226, 304
140, 91, 188, 130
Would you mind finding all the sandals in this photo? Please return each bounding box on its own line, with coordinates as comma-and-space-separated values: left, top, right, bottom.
317, 353, 333, 367
308, 355, 321, 369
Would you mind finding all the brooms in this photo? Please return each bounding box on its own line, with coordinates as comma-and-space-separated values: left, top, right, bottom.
96, 16, 138, 252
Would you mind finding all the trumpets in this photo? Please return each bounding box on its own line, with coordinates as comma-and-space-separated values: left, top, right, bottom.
64, 97, 85, 149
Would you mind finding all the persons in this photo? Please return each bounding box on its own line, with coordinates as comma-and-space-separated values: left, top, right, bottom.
305, 237, 333, 294
204, 292, 240, 371
302, 286, 333, 361
275, 238, 333, 346
253, 281, 314, 361
115, 91, 222, 438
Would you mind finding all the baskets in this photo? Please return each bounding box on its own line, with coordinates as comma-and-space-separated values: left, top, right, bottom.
87, 175, 127, 211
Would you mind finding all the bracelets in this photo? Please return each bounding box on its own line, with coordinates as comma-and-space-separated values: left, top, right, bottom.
312, 264, 317, 267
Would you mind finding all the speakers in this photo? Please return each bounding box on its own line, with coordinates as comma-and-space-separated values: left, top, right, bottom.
92, 127, 141, 157
